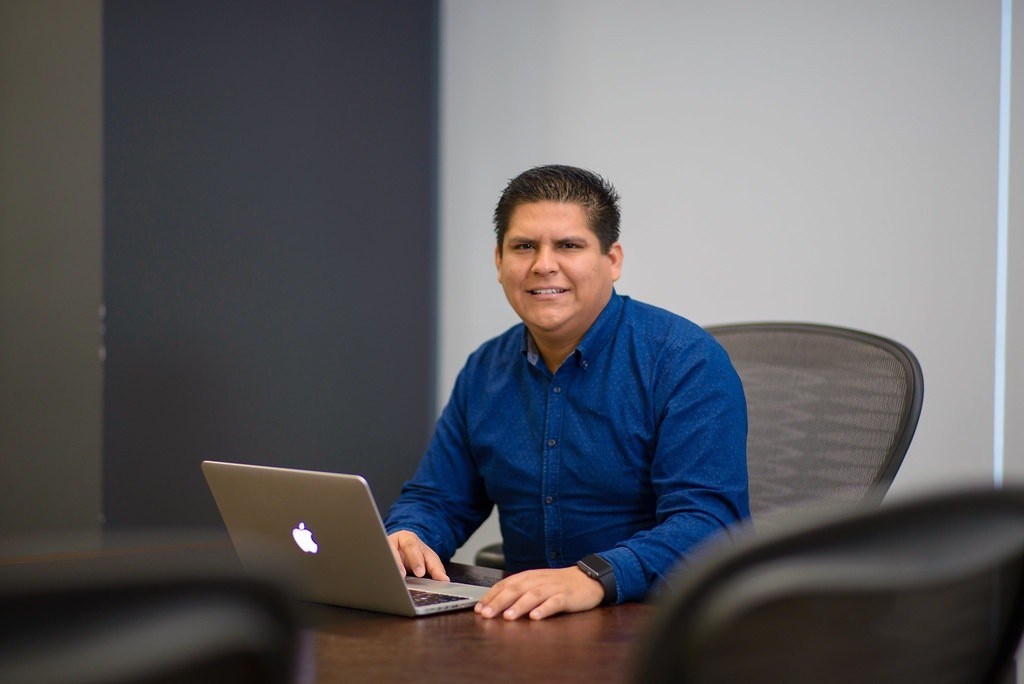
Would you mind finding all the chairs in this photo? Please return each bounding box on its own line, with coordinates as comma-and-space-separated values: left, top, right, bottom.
696, 321, 924, 546
619, 479, 1024, 684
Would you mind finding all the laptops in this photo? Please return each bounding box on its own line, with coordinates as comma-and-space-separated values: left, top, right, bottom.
201, 460, 492, 617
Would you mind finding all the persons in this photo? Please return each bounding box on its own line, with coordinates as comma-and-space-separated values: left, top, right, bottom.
383, 165, 751, 621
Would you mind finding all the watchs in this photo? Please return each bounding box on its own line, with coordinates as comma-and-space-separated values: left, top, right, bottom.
577, 553, 617, 607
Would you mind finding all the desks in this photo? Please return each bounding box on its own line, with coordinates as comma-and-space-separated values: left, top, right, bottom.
299, 562, 667, 684
